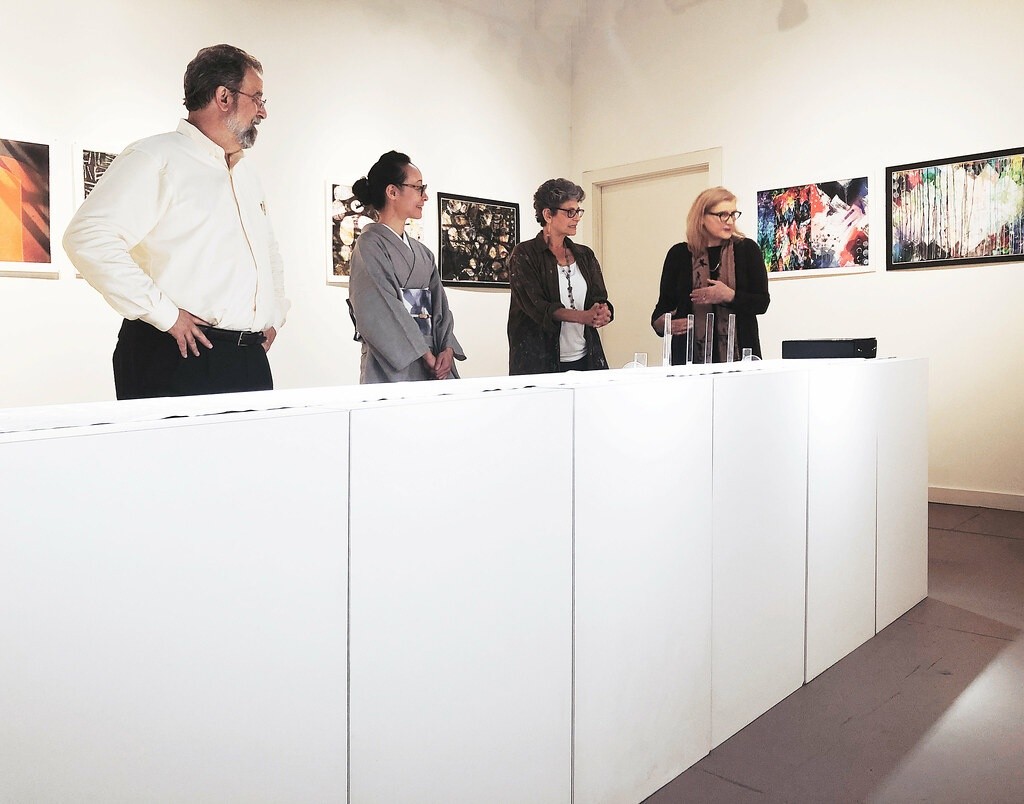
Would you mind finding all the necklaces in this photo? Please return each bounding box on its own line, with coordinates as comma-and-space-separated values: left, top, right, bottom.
709, 262, 721, 272
548, 241, 577, 310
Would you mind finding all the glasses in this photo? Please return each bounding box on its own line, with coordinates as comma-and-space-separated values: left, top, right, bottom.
399, 183, 427, 197
554, 207, 584, 218
226, 87, 267, 108
709, 211, 742, 223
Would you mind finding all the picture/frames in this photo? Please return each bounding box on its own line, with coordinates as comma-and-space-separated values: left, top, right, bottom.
0, 132, 123, 279
324, 176, 428, 282
439, 191, 521, 288
886, 146, 1024, 270
753, 171, 877, 277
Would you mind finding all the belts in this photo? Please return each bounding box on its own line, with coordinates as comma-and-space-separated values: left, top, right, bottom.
194, 325, 268, 348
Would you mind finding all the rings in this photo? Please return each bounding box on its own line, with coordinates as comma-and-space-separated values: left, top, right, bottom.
703, 297, 706, 301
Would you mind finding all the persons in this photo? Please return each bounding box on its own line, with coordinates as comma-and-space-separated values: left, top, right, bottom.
651, 188, 770, 366
346, 151, 467, 386
505, 177, 614, 376
64, 43, 290, 401
